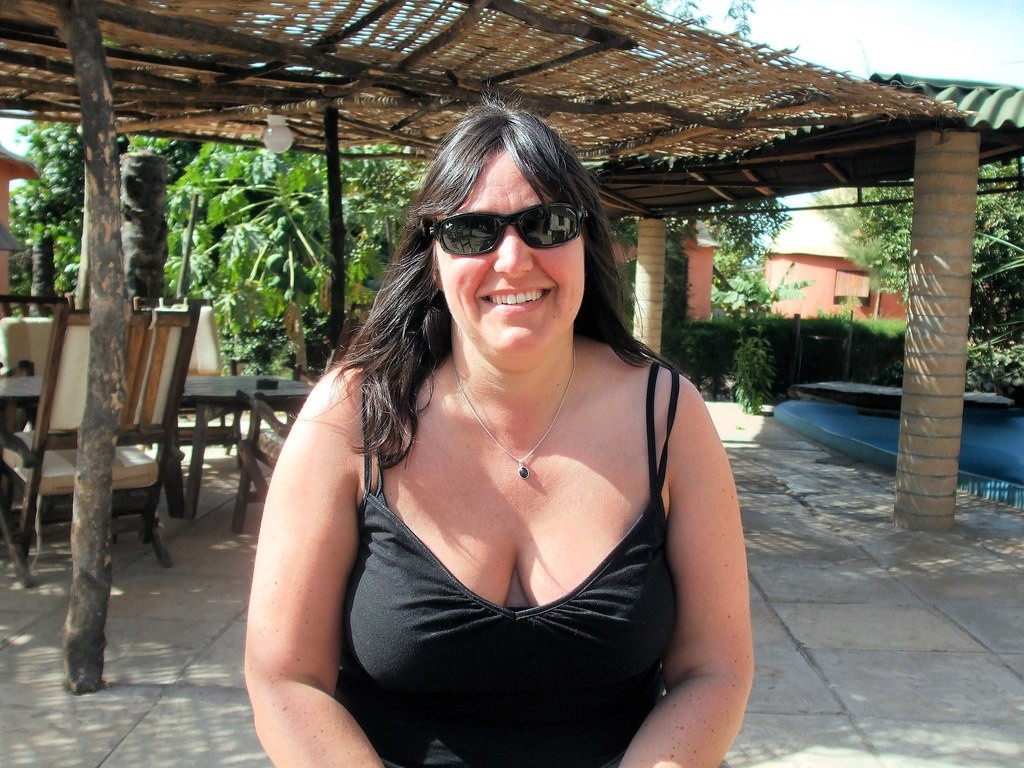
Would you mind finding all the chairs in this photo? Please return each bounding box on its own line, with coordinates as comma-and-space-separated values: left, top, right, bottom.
0, 297, 204, 589
0, 291, 76, 432
131, 295, 245, 468
225, 301, 373, 533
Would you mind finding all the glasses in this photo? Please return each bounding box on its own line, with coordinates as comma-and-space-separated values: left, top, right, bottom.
429, 203, 589, 256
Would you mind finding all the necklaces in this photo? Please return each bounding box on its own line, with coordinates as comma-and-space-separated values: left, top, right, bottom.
452, 345, 576, 480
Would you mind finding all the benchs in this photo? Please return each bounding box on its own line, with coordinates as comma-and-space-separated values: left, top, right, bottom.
794, 380, 1016, 420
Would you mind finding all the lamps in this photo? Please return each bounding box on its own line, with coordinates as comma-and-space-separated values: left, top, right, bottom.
263, 113, 295, 154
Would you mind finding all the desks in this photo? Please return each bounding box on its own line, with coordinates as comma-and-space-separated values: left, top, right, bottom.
0, 376, 314, 519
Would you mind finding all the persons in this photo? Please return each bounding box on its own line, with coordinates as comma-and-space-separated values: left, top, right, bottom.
242, 103, 753, 768
